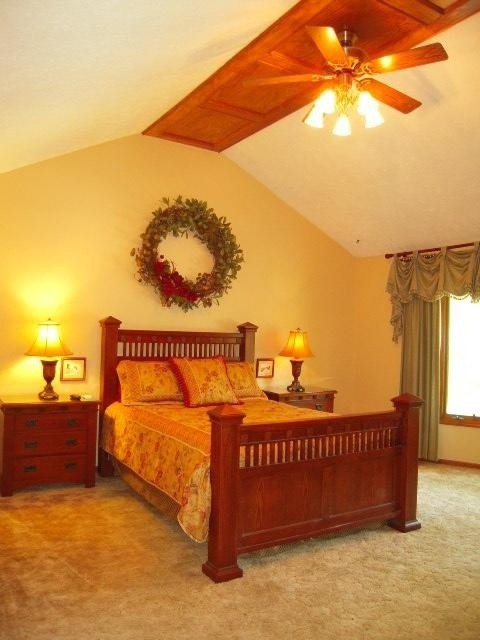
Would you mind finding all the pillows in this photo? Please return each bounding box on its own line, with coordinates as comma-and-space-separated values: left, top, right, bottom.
117, 357, 266, 411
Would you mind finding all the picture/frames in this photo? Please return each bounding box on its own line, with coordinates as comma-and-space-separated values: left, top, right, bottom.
255, 358, 275, 378
60, 356, 88, 382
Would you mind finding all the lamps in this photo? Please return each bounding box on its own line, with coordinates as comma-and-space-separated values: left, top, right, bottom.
25, 322, 72, 399
279, 328, 315, 393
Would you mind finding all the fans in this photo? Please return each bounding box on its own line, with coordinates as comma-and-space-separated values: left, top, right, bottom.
239, 24, 449, 116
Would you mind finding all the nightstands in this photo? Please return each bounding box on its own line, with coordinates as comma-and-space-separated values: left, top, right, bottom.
265, 384, 337, 414
1, 396, 102, 498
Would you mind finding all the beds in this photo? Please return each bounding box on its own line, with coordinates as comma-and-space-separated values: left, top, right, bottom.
97, 313, 425, 580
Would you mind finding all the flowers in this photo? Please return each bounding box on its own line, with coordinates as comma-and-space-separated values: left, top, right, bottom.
128, 193, 242, 310
